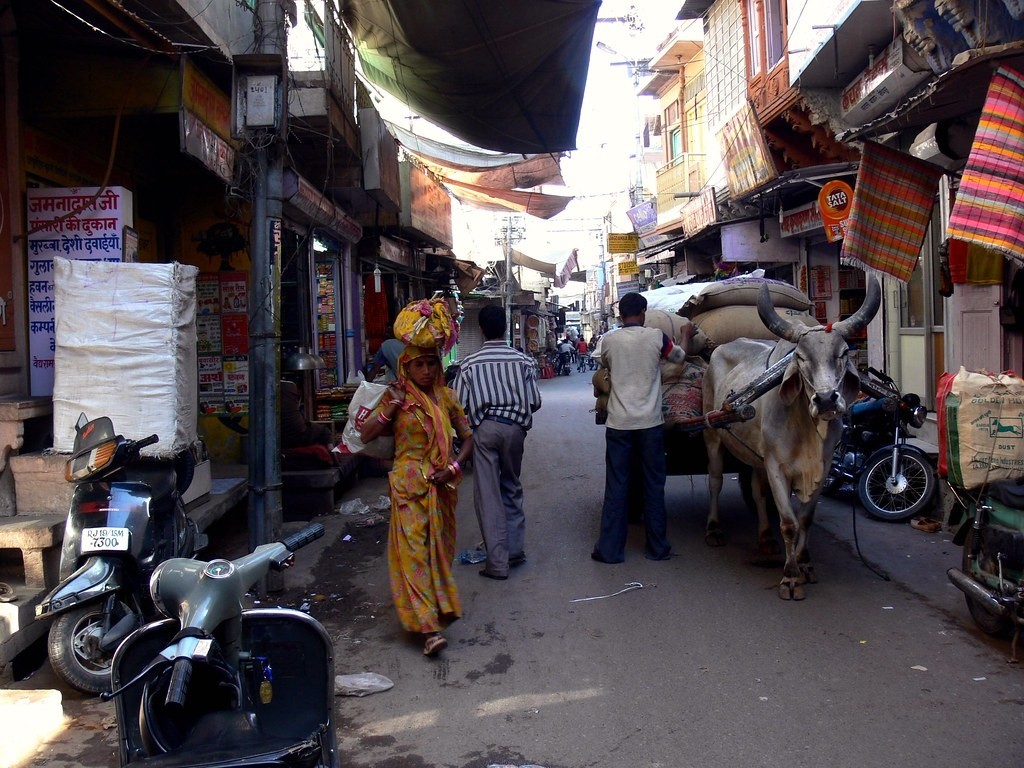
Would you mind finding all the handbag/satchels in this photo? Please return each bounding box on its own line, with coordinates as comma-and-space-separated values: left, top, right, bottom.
332, 381, 396, 458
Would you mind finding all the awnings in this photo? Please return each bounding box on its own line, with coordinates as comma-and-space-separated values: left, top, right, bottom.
525, 307, 555, 317
835, 38, 1024, 147
728, 160, 862, 241
645, 214, 770, 260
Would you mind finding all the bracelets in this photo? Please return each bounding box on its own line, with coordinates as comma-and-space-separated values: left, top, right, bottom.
389, 400, 404, 407
447, 460, 460, 476
376, 411, 391, 426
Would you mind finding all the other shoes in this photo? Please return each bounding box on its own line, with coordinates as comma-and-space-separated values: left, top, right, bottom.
479, 569, 508, 580
424, 635, 448, 655
509, 552, 526, 565
591, 551, 616, 564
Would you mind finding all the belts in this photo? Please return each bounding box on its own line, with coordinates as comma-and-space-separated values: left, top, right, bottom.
485, 416, 513, 425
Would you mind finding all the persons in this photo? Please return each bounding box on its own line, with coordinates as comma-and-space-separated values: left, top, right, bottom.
591, 292, 700, 564
365, 338, 406, 499
453, 305, 542, 582
555, 333, 603, 376
359, 341, 476, 656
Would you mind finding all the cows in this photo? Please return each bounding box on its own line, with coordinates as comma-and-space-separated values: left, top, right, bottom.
701, 273, 882, 601
